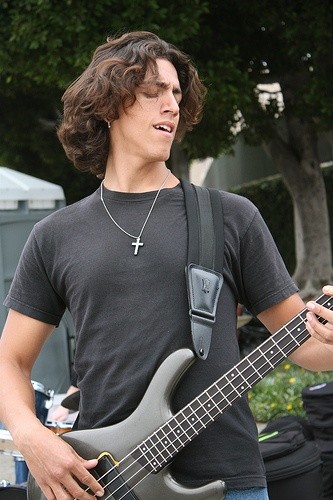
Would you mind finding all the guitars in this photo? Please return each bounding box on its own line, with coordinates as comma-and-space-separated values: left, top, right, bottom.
26, 283, 332, 500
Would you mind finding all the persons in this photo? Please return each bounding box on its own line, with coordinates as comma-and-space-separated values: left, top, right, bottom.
0, 30, 333, 500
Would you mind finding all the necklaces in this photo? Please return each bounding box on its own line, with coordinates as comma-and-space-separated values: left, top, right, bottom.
100, 168, 170, 255
